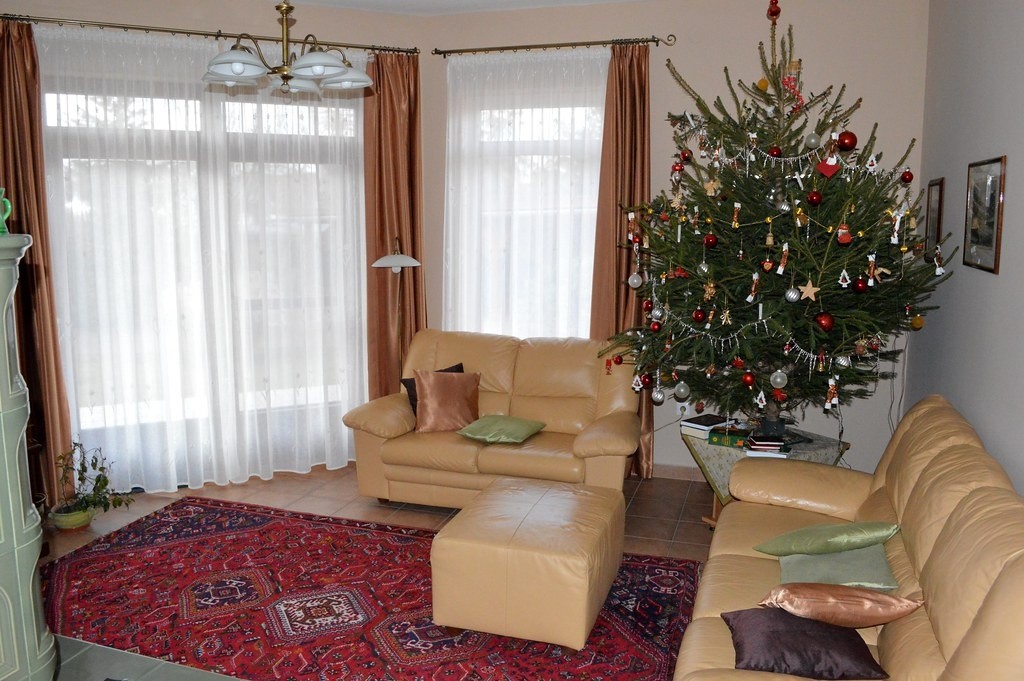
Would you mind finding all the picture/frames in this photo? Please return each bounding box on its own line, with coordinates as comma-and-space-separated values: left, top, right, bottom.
924, 177, 944, 263
962, 155, 1006, 275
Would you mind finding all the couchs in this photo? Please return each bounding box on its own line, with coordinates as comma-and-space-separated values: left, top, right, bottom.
342, 329, 642, 510
674, 395, 1024, 681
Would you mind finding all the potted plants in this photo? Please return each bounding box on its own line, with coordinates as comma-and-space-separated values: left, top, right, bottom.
50, 442, 136, 534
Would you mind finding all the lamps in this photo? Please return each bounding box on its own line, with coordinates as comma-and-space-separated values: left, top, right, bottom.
371, 237, 421, 393
202, 0, 373, 93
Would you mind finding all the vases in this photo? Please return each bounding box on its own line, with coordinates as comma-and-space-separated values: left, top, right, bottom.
32, 492, 47, 527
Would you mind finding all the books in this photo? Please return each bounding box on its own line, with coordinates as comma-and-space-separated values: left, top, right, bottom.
777, 429, 805, 446
679, 413, 736, 440
708, 426, 754, 449
743, 435, 793, 459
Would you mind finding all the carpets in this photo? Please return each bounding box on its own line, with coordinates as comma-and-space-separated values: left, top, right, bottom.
39, 497, 703, 681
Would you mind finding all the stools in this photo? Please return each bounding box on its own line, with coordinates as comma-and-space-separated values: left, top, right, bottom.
430, 475, 626, 650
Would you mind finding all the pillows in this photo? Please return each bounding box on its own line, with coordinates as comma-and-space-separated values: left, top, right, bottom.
400, 362, 464, 432
752, 521, 900, 557
456, 414, 547, 444
720, 607, 890, 681
758, 583, 925, 628
412, 368, 481, 432
777, 543, 898, 593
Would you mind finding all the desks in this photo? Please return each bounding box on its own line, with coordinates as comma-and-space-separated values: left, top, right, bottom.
682, 422, 850, 531
